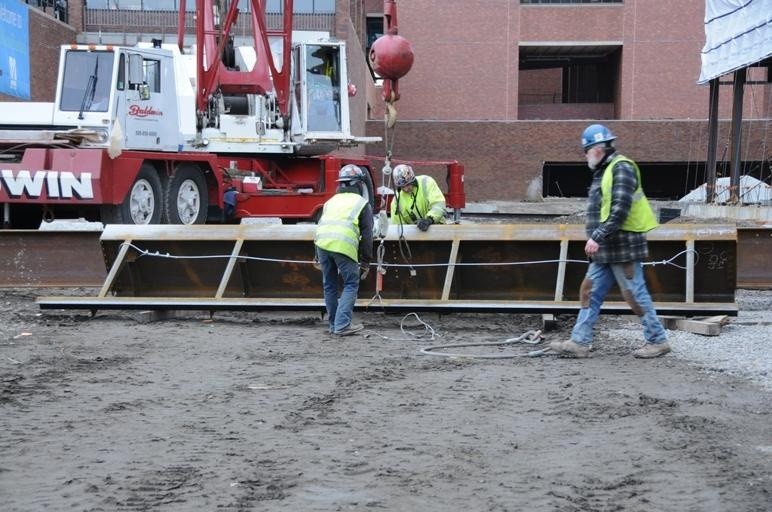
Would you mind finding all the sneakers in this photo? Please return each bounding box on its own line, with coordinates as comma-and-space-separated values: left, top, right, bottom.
633, 342, 671, 359
338, 323, 365, 334
550, 339, 589, 358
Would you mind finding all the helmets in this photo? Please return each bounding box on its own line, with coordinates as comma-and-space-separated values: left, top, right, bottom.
580, 123, 617, 148
335, 163, 367, 182
392, 164, 415, 187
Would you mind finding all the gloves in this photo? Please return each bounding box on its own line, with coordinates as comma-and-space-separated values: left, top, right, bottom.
359, 259, 370, 281
414, 216, 434, 232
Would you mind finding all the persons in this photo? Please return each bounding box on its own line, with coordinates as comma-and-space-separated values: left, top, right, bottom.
314, 164, 374, 336
547, 123, 672, 359
390, 164, 448, 232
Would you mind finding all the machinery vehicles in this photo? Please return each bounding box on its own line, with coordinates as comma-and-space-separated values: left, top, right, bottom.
0, 0, 466, 230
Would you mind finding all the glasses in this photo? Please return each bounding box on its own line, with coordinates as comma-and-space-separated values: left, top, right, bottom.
584, 147, 592, 154
